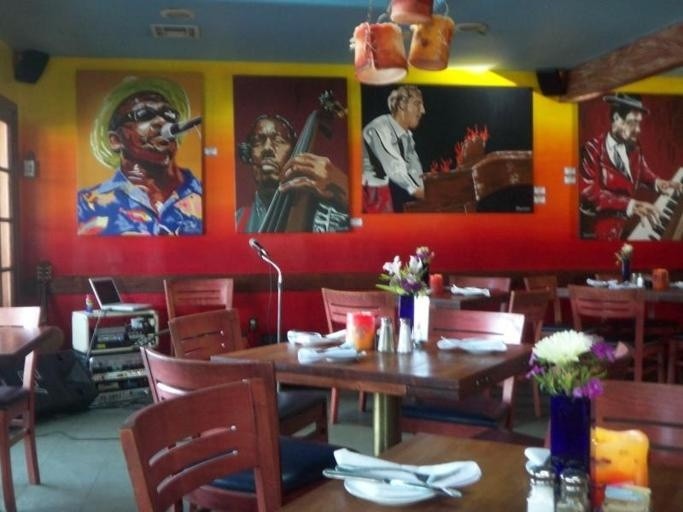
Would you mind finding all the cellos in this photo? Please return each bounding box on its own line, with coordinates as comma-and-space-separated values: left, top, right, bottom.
259, 87, 348, 232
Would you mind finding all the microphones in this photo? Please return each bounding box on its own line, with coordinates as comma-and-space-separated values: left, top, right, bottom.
249, 238, 268, 256
247, 155, 262, 169
160, 115, 202, 139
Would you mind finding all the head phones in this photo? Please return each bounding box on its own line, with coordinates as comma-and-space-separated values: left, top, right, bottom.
239, 113, 295, 160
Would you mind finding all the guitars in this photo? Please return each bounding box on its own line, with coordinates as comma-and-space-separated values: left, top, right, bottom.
14, 260, 65, 359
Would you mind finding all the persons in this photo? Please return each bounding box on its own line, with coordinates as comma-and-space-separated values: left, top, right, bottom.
363, 88, 432, 213
573, 95, 679, 240
234, 109, 348, 235
77, 73, 200, 235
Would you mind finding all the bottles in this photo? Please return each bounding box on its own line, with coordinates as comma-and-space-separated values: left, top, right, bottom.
396, 318, 410, 355
553, 468, 592, 512
631, 273, 645, 289
85, 293, 94, 312
524, 463, 555, 511
376, 316, 395, 354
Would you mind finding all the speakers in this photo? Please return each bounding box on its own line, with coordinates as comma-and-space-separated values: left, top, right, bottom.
15, 50, 49, 84
536, 68, 568, 96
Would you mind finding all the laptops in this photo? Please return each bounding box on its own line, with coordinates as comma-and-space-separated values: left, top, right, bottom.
88, 277, 152, 311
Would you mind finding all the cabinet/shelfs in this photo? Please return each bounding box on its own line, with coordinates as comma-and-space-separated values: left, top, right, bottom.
66, 307, 163, 384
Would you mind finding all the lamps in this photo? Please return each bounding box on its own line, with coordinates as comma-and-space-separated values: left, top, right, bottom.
346, 0, 455, 90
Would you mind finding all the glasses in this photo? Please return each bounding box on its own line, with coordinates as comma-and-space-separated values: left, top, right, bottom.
112, 107, 180, 130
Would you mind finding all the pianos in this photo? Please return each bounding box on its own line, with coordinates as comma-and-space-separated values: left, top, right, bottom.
627, 167, 683, 241
402, 150, 534, 214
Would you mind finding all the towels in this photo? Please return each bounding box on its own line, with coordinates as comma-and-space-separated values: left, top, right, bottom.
321, 444, 487, 499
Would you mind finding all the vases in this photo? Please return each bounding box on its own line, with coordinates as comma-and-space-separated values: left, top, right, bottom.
544, 392, 596, 477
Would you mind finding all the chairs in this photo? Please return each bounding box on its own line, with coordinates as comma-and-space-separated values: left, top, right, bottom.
137, 341, 369, 511
116, 374, 281, 510
166, 307, 332, 445
158, 269, 682, 383
578, 376, 683, 467
0, 306, 44, 511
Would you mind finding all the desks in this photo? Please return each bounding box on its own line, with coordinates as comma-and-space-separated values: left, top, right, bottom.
0, 324, 57, 364
208, 335, 536, 460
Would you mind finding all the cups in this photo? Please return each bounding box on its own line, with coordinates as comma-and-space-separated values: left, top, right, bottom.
428, 274, 443, 297
348, 312, 375, 351
652, 267, 669, 289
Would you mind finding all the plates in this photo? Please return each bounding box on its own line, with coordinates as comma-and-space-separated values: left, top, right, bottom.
436, 336, 507, 355
317, 446, 482, 506
284, 327, 367, 364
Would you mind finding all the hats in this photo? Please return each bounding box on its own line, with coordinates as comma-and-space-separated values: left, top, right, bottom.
90, 77, 190, 169
603, 93, 649, 111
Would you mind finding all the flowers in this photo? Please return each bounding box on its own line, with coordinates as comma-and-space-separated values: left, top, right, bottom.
372, 242, 435, 294
